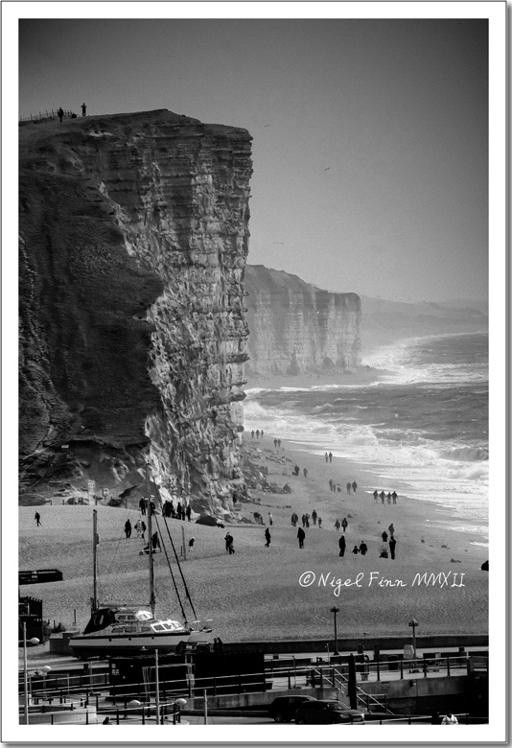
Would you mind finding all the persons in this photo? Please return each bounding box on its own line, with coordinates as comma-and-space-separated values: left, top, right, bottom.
292, 463, 309, 478
34, 510, 42, 526
329, 478, 360, 493
371, 487, 400, 505
224, 531, 235, 554
103, 717, 113, 725
430, 710, 459, 725
251, 429, 266, 438
123, 495, 196, 558
273, 437, 281, 448
252, 509, 397, 560
325, 451, 333, 463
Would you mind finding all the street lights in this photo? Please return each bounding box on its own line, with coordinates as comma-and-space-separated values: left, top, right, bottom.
329, 605, 340, 655
23, 621, 52, 725
408, 617, 419, 658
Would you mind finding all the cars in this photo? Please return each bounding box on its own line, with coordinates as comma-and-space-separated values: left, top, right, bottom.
267, 694, 365, 724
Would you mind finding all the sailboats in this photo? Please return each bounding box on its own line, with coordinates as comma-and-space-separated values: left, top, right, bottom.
68, 458, 213, 661
